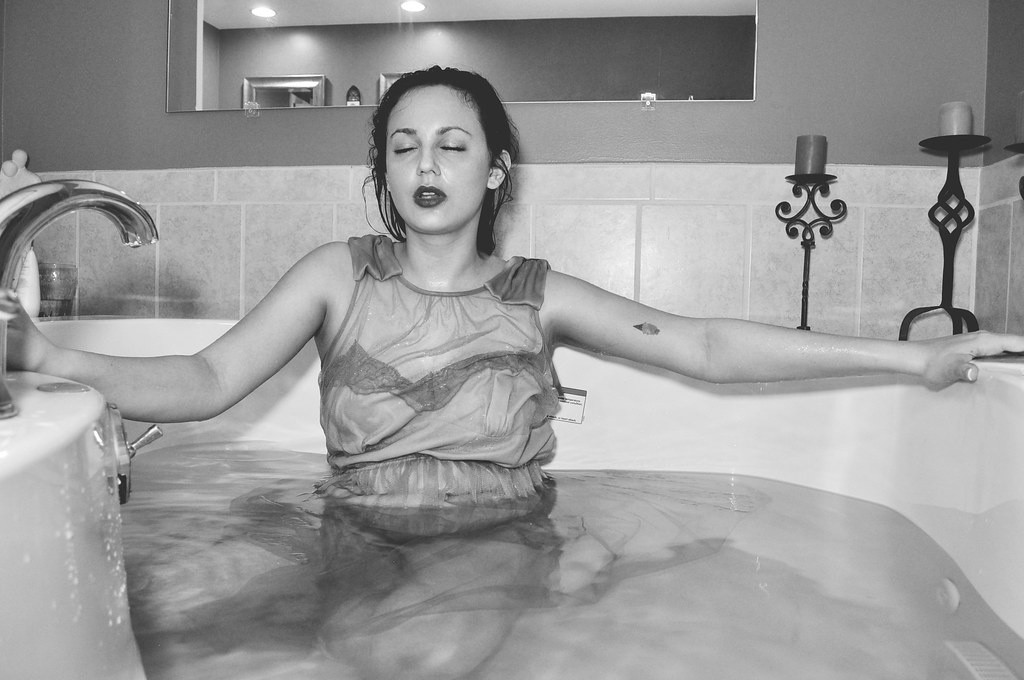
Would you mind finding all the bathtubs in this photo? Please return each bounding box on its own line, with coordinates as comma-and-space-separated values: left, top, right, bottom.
0, 312, 1024, 680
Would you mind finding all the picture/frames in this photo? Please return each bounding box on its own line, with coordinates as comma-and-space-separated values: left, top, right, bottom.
243, 74, 325, 109
380, 71, 415, 104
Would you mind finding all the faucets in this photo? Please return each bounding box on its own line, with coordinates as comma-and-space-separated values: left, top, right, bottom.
0, 177, 160, 420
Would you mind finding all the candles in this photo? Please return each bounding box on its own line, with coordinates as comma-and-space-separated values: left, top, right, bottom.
796, 133, 826, 173
937, 99, 973, 134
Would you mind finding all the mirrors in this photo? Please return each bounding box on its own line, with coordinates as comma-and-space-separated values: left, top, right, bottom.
166, 0, 759, 111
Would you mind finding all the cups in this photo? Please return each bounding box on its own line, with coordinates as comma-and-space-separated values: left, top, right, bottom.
37, 262, 77, 317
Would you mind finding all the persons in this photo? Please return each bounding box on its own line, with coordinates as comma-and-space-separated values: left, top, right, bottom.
0, 67, 1024, 509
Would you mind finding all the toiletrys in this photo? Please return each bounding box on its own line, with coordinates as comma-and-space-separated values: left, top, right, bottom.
15, 244, 41, 317
